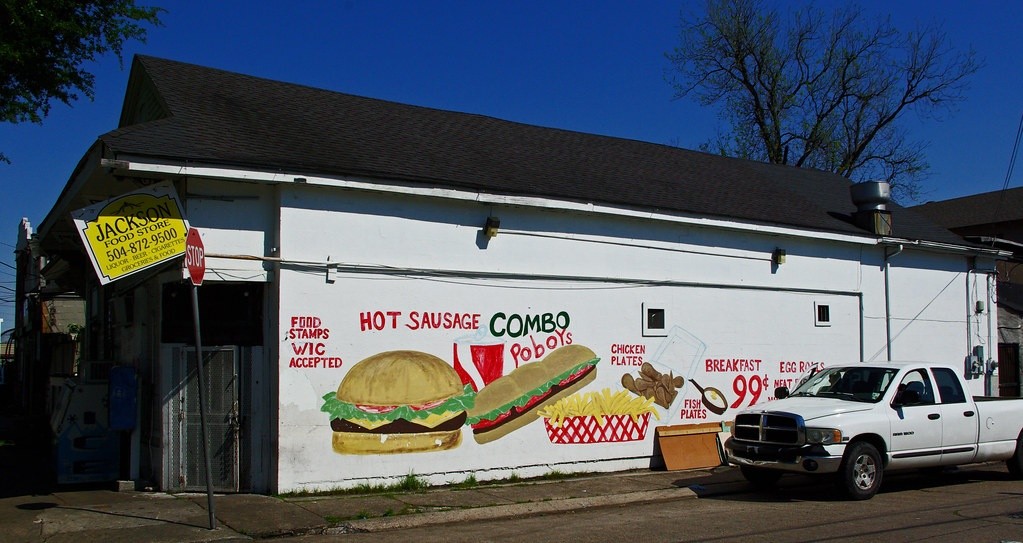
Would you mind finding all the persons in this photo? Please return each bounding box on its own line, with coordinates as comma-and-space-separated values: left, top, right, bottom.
816, 371, 841, 392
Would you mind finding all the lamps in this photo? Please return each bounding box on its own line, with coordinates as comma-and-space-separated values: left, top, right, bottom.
773, 249, 786, 264
484, 216, 500, 236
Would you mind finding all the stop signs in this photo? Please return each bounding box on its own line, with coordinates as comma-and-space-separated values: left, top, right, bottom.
184, 228, 205, 288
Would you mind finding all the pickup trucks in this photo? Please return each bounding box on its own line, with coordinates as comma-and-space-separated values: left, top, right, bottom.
724, 359, 1022, 500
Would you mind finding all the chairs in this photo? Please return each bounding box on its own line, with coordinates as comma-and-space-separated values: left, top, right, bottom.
905, 381, 923, 401
853, 381, 875, 393
